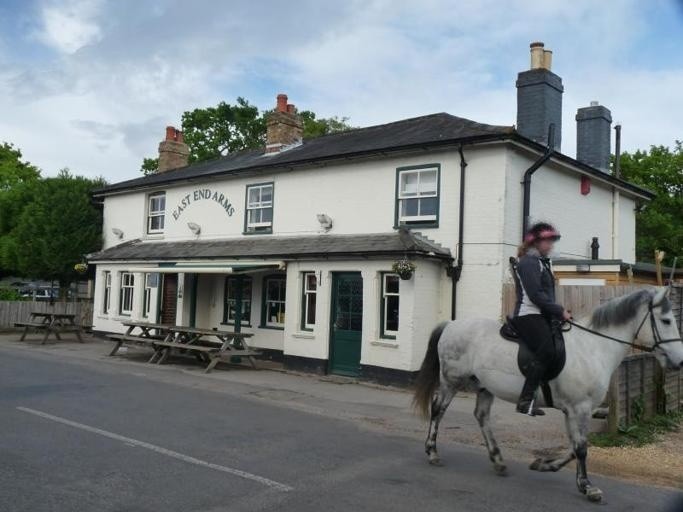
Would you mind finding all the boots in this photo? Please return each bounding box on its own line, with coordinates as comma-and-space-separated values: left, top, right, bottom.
514, 359, 546, 418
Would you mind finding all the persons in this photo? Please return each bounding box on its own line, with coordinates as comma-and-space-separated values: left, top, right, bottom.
508, 223, 573, 417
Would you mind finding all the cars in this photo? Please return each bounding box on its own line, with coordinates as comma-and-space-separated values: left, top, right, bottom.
3, 279, 75, 301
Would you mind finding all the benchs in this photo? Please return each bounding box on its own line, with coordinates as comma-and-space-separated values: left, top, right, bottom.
14, 320, 47, 329
82, 324, 95, 329
106, 333, 263, 359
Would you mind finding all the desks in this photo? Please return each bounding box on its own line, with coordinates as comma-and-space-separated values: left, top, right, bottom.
110, 321, 259, 373
20, 312, 82, 344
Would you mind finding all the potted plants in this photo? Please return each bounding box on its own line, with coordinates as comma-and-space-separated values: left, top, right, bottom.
393, 253, 415, 280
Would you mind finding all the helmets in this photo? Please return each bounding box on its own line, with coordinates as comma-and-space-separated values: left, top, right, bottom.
521, 222, 563, 243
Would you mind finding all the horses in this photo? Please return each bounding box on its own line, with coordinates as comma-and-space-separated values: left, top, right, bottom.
412, 281, 683, 503
